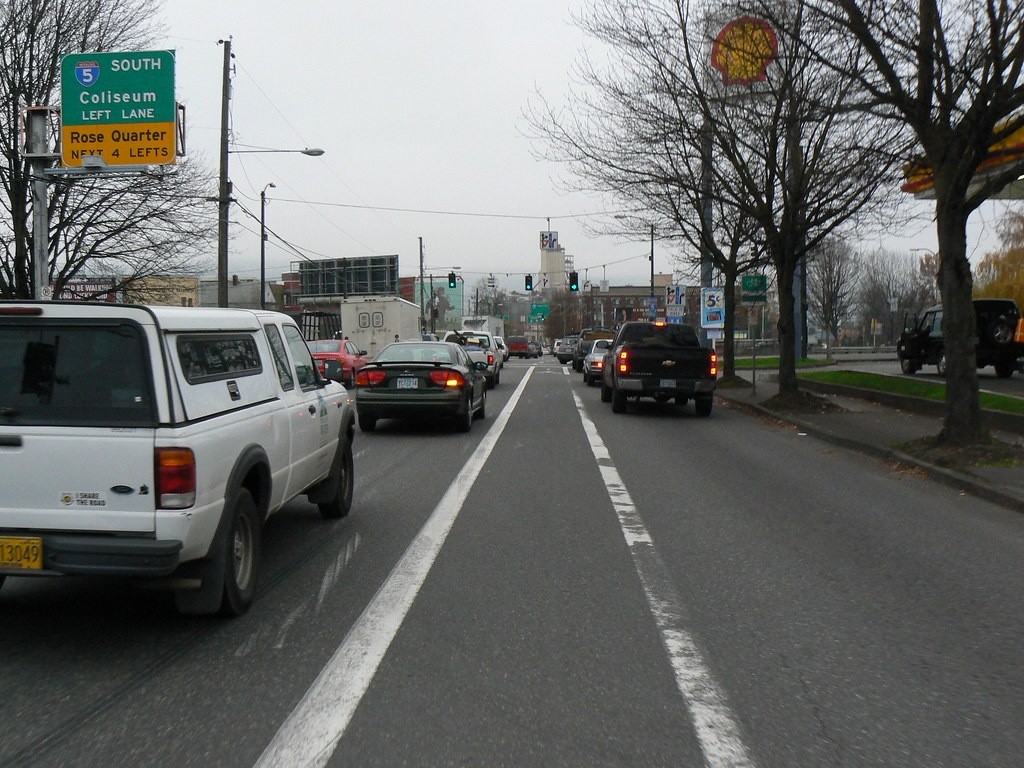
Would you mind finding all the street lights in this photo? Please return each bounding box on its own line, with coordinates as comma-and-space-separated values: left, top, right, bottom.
218, 150, 323, 306
420, 267, 462, 333
911, 248, 938, 302
614, 215, 655, 321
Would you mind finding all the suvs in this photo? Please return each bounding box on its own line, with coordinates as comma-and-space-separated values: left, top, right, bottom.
506, 336, 530, 359
895, 299, 1023, 379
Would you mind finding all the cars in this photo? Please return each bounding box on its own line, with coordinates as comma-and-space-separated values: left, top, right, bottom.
423, 333, 439, 343
528, 335, 580, 364
494, 337, 509, 366
582, 340, 615, 385
303, 338, 367, 386
354, 342, 488, 432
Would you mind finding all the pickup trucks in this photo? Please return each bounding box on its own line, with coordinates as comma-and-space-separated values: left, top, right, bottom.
433, 332, 504, 389
596, 321, 718, 413
574, 329, 617, 372
0, 304, 355, 619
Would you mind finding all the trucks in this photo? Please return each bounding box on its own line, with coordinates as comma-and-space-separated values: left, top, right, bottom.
341, 297, 426, 360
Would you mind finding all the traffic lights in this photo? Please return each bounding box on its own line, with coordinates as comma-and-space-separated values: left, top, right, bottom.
449, 273, 456, 288
524, 275, 532, 290
570, 273, 579, 291
